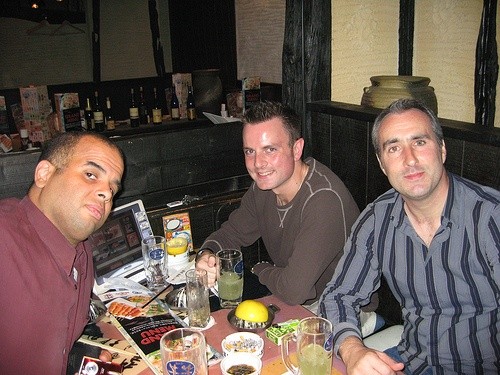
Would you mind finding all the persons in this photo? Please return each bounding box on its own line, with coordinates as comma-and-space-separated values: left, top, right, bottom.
0, 129, 125, 375
317, 99, 500, 375
195, 99, 380, 330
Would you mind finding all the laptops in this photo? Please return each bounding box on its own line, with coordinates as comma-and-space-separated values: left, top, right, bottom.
85, 199, 158, 299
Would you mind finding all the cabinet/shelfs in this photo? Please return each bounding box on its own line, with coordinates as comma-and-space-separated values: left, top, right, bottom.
0, 114, 254, 212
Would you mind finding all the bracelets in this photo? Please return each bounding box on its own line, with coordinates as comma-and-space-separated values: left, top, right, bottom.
195, 247, 214, 267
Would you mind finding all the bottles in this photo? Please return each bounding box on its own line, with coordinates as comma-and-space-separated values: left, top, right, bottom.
152, 88, 163, 125
129, 88, 139, 128
170, 86, 180, 121
106, 96, 115, 131
84, 97, 94, 132
138, 86, 149, 124
191, 68, 223, 119
186, 85, 196, 121
93, 91, 104, 132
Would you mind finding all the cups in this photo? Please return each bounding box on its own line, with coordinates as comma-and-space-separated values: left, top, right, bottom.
141, 236, 169, 291
220, 353, 262, 375
185, 269, 211, 328
281, 316, 333, 375
167, 247, 190, 266
216, 249, 244, 309
160, 328, 208, 375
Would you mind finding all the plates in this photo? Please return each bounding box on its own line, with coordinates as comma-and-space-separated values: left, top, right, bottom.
166, 262, 195, 285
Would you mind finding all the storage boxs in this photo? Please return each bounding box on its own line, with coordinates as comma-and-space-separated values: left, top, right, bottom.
267, 318, 302, 346
163, 212, 193, 252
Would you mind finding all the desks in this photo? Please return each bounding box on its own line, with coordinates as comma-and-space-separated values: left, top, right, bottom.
171, 265, 348, 375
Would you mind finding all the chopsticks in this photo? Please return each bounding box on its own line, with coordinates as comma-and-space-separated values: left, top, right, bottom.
168, 258, 195, 283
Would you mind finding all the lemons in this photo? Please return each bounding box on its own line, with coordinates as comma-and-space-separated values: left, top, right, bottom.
236, 300, 269, 323
166, 238, 188, 254
